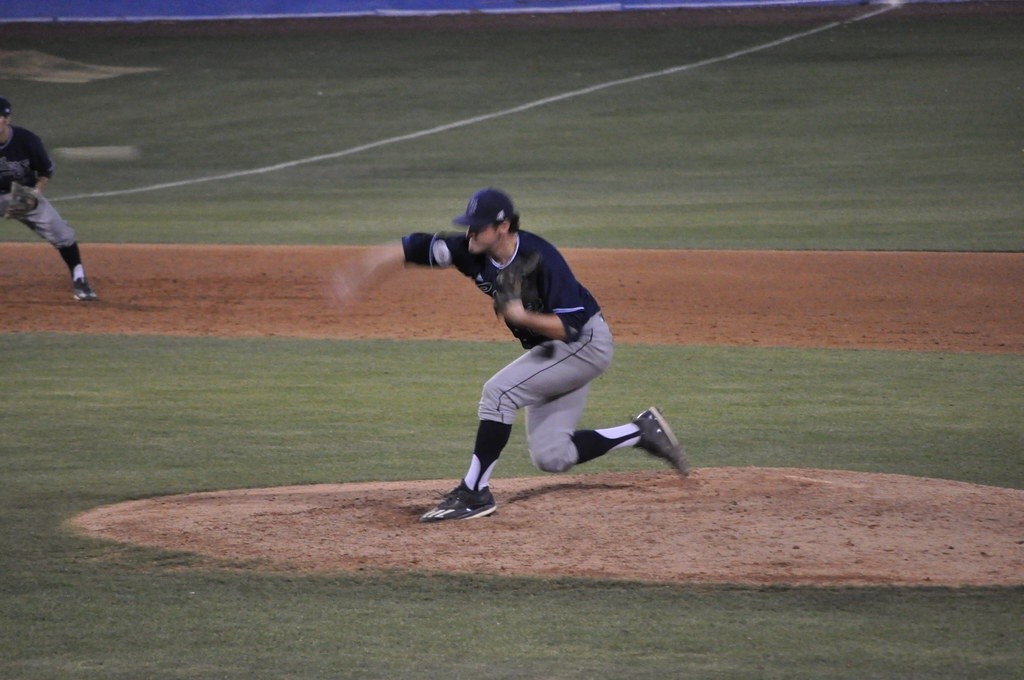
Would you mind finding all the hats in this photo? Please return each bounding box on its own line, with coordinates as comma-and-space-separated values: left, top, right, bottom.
0, 97, 11, 116
453, 188, 513, 226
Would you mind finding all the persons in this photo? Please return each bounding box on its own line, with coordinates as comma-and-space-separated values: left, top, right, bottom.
0, 98, 97, 301
358, 188, 688, 522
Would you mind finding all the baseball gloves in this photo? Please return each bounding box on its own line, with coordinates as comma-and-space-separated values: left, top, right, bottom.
4, 181, 47, 220
494, 264, 546, 322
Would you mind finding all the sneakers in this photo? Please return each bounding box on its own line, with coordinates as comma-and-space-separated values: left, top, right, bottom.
72, 277, 96, 301
633, 406, 690, 477
419, 479, 496, 523
